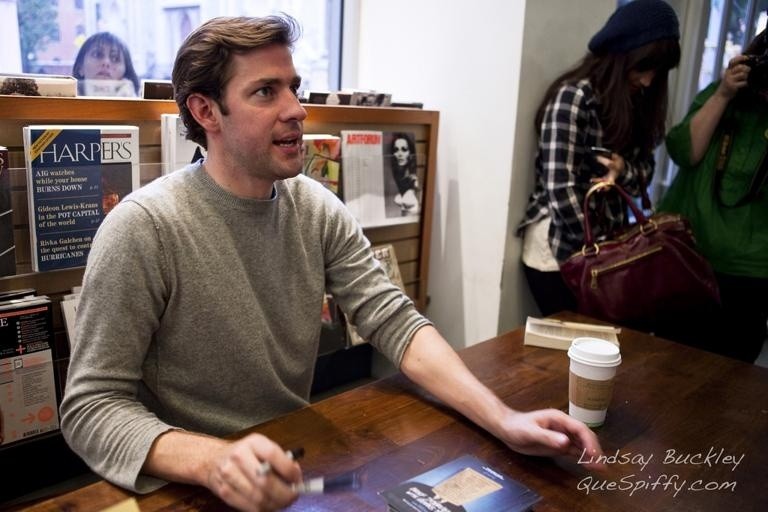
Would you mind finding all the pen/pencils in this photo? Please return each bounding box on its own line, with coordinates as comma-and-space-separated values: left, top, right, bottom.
302, 476, 354, 494
256, 447, 304, 478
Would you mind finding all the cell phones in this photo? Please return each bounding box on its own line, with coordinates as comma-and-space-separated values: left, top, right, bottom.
590, 145, 611, 158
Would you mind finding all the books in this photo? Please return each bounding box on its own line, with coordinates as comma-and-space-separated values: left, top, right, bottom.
141, 77, 176, 100
523, 312, 624, 357
377, 454, 545, 509
297, 90, 428, 349
159, 110, 212, 175
0, 148, 19, 277
63, 285, 90, 348
1, 74, 80, 96
21, 119, 145, 272
1, 291, 63, 444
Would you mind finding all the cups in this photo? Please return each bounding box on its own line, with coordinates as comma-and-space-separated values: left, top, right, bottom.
567, 335, 623, 430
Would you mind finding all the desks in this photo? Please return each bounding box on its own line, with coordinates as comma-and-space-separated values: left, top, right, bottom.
17, 309, 768, 512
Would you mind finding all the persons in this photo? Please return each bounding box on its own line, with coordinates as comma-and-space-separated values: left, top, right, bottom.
523, 0, 675, 341
70, 30, 140, 94
61, 11, 606, 510
643, 26, 767, 369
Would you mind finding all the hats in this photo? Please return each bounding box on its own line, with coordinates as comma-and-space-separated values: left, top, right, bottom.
588, 0, 681, 52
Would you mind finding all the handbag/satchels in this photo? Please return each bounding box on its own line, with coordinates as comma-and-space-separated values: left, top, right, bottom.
560, 212, 718, 323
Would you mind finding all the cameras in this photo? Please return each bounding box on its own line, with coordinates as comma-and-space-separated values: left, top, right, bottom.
745, 56, 768, 94
